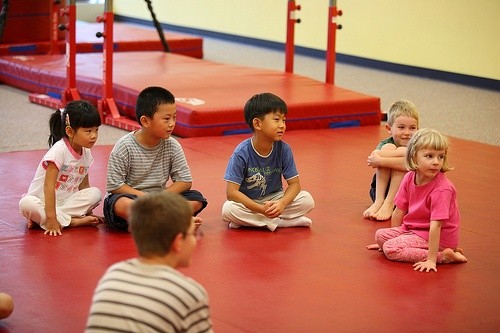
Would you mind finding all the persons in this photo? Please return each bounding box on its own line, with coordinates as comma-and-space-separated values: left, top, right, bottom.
103, 86, 209, 236
363, 99, 424, 222
0, 293, 14, 318
84, 191, 214, 333
19, 100, 105, 237
365, 128, 467, 272
220, 92, 316, 233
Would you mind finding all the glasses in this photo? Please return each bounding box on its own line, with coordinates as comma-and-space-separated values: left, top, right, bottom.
186, 228, 203, 241
